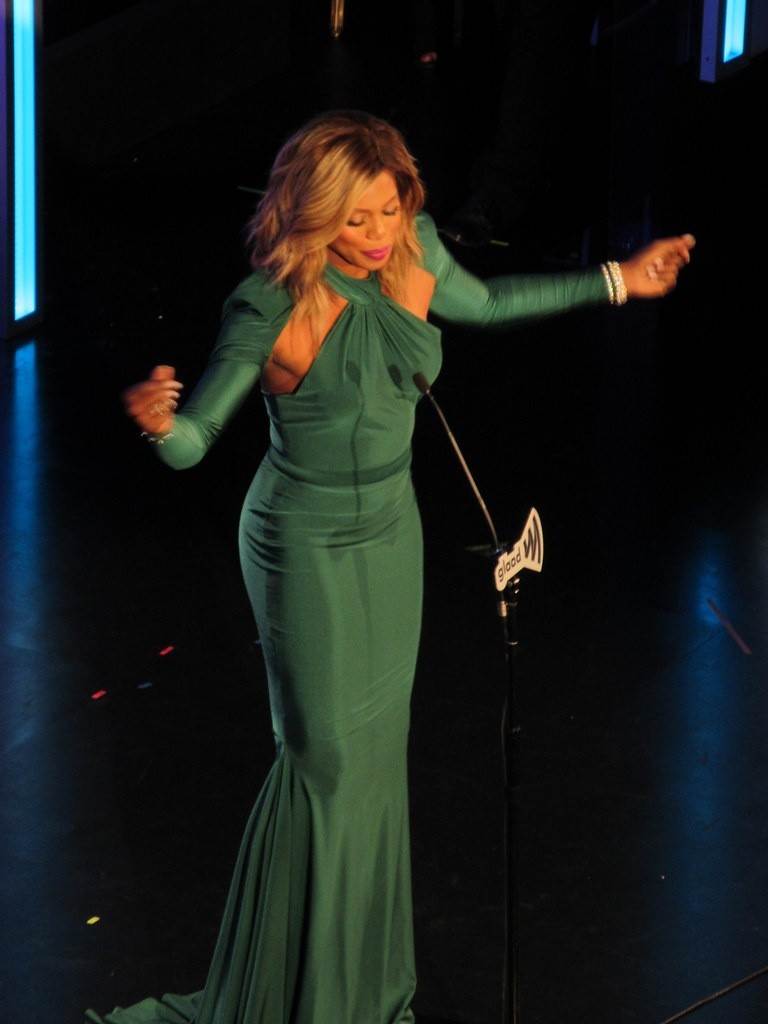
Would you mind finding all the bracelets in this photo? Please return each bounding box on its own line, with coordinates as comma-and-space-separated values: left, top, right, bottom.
601, 263, 615, 306
607, 259, 628, 306
141, 431, 175, 443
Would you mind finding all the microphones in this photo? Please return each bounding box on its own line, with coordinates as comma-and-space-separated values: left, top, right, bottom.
412, 370, 501, 554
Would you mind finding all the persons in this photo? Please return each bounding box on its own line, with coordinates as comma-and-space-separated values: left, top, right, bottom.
125, 113, 699, 1024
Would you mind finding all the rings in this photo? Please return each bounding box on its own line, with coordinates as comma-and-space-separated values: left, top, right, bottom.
149, 401, 168, 416
